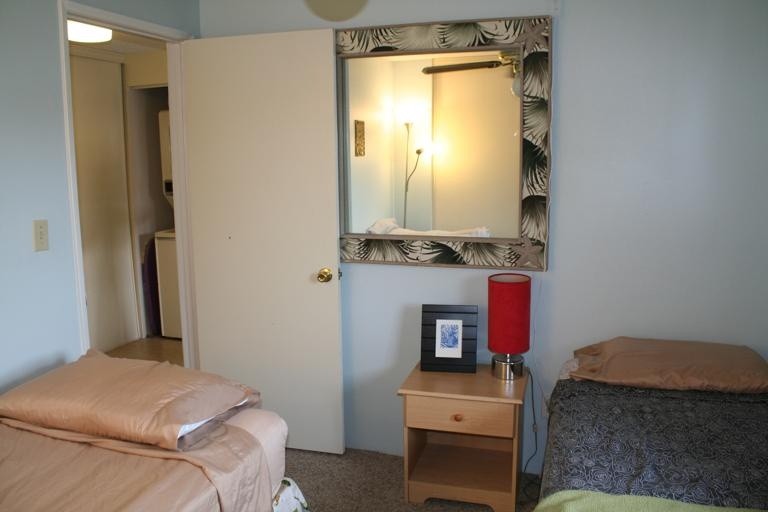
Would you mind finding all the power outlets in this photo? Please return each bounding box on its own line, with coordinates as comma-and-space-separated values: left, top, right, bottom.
32, 218, 49, 253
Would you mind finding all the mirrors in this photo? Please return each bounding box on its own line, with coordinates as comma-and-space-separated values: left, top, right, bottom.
335, 15, 553, 274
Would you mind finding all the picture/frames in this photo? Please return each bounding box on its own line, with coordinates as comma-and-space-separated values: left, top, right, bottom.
421, 304, 477, 374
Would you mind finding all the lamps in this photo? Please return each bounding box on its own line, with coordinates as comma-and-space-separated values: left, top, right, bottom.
486, 273, 529, 379
388, 102, 447, 230
67, 19, 117, 43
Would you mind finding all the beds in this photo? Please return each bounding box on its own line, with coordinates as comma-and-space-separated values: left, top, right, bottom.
1, 347, 310, 511
542, 337, 768, 512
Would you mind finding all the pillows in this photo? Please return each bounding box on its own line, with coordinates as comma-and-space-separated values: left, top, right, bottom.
0, 347, 264, 450
571, 337, 768, 393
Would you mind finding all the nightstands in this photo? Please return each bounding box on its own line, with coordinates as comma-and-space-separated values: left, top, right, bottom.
397, 356, 528, 512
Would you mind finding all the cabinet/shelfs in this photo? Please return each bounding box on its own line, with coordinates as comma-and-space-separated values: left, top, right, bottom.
155, 231, 181, 339
